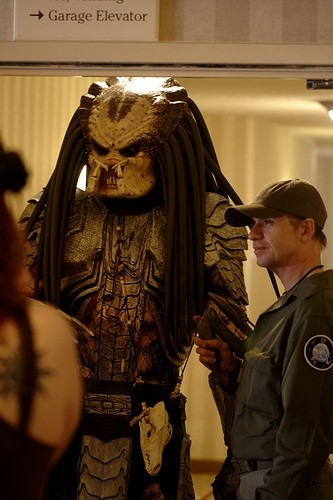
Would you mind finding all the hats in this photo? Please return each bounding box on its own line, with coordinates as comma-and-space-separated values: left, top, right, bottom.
224, 178, 327, 229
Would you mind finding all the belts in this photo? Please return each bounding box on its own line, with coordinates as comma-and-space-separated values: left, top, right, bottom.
231, 458, 273, 473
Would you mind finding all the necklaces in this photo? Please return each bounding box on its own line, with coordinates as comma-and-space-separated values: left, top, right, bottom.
283, 265, 325, 295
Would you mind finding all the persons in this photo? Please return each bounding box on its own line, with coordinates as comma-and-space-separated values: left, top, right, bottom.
192, 178, 333, 500
0, 195, 84, 500
18, 75, 280, 500
